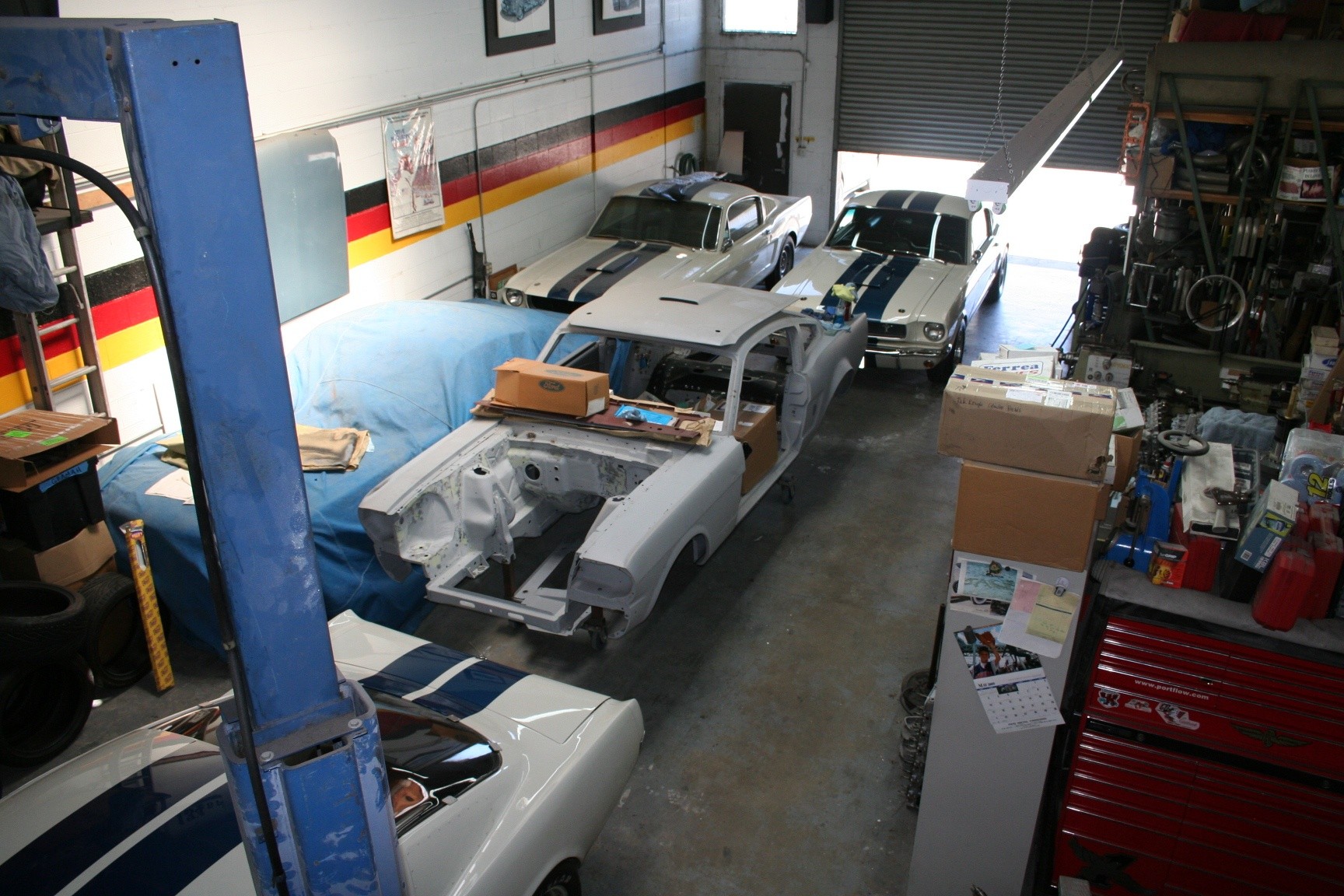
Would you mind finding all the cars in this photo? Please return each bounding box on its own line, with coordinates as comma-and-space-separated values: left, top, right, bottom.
767, 188, 1010, 390
1, 607, 645, 896
501, 169, 812, 326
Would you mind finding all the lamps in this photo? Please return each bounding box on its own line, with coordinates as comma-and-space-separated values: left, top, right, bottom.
965, 0, 1124, 217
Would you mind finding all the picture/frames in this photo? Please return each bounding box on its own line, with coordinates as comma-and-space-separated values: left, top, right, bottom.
593, 0, 645, 35
484, 0, 556, 56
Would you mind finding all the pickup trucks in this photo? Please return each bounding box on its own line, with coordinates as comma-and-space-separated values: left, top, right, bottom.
359, 279, 869, 653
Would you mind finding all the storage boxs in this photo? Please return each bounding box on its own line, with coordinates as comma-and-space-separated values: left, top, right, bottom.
0, 408, 120, 588
491, 356, 609, 417
1296, 326, 1340, 413
1234, 477, 1298, 567
687, 398, 776, 494
938, 345, 1140, 572
1276, 159, 1335, 204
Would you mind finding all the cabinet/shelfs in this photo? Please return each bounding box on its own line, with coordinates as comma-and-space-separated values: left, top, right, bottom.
1144, 108, 1344, 210
